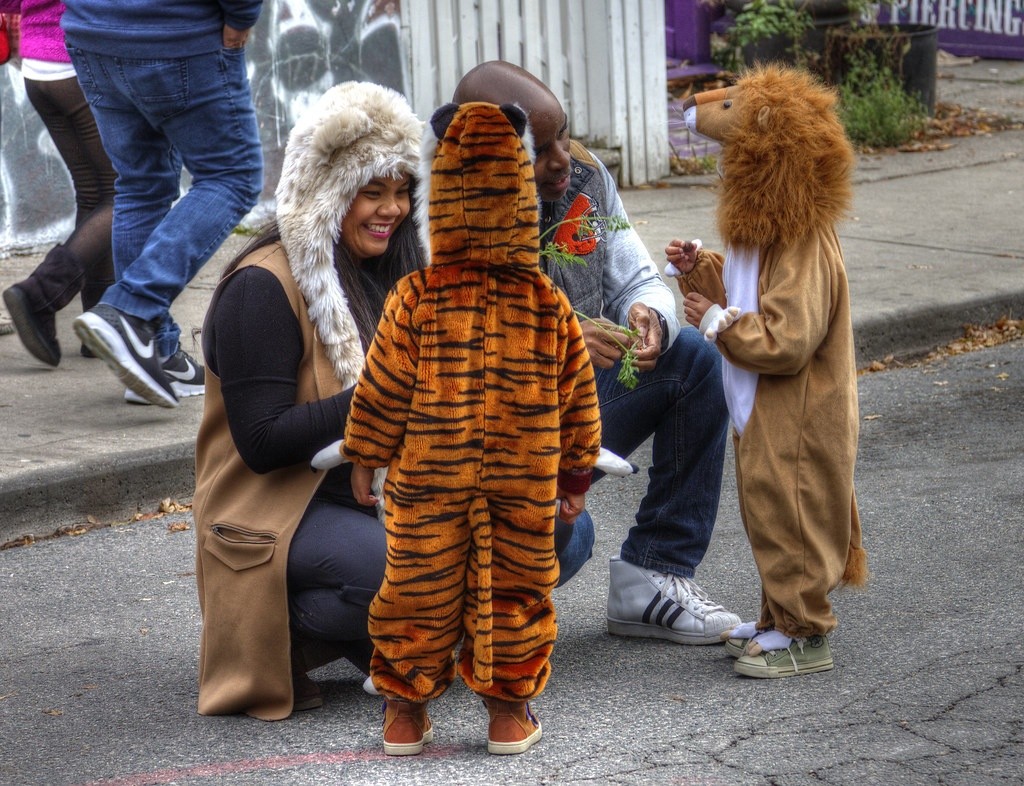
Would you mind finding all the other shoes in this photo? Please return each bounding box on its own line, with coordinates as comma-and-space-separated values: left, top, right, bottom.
286, 667, 324, 712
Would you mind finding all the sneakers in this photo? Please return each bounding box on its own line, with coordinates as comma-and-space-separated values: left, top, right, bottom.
718, 617, 771, 657
125, 344, 205, 403
379, 700, 433, 754
605, 554, 742, 644
74, 301, 179, 409
480, 695, 542, 755
732, 635, 833, 678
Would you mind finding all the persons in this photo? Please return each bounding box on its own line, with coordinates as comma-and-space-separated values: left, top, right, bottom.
0, 0, 265, 409
435, 60, 741, 647
664, 64, 868, 678
191, 79, 593, 721
346, 102, 601, 756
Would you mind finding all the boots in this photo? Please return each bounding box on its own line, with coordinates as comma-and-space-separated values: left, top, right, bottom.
77, 278, 117, 357
3, 241, 94, 366
